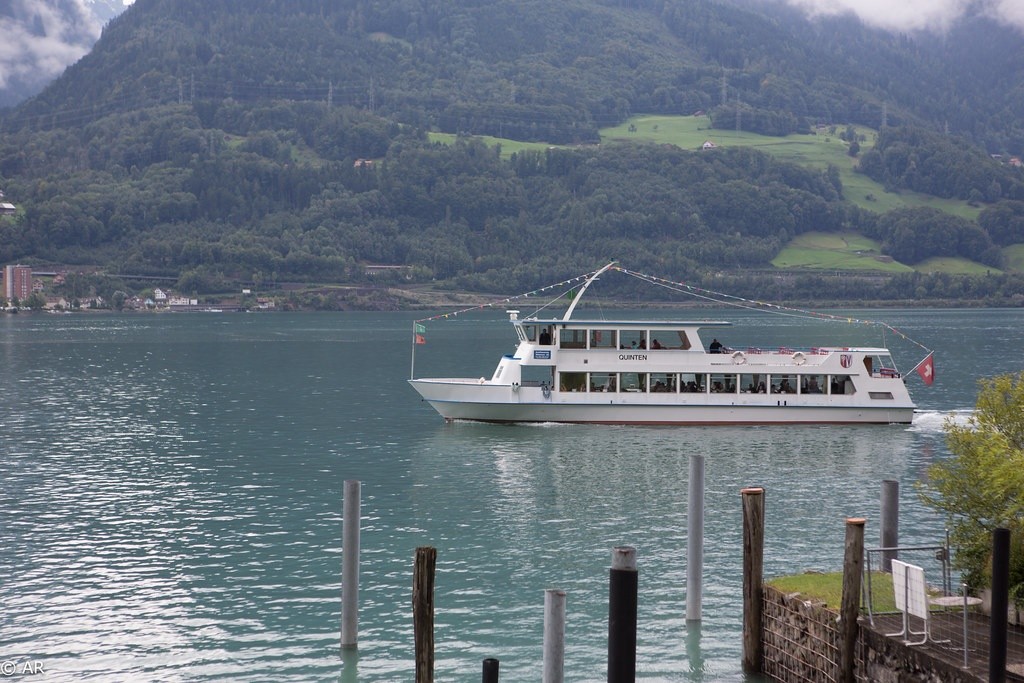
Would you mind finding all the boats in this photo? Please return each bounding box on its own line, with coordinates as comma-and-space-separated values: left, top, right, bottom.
199, 307, 223, 313
406, 259, 917, 423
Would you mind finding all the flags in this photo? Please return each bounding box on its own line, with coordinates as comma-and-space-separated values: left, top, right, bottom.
915, 354, 935, 385
416, 335, 425, 344
415, 323, 425, 333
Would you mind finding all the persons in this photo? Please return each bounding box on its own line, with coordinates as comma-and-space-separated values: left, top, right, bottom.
630, 340, 638, 349
641, 381, 703, 392
709, 338, 722, 354
772, 376, 839, 395
560, 373, 617, 392
652, 339, 660, 349
712, 380, 766, 393
639, 339, 646, 349
615, 341, 624, 349
539, 329, 551, 345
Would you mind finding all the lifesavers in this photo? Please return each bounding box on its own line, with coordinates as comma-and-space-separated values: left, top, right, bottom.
792, 351, 806, 365
731, 351, 746, 365
